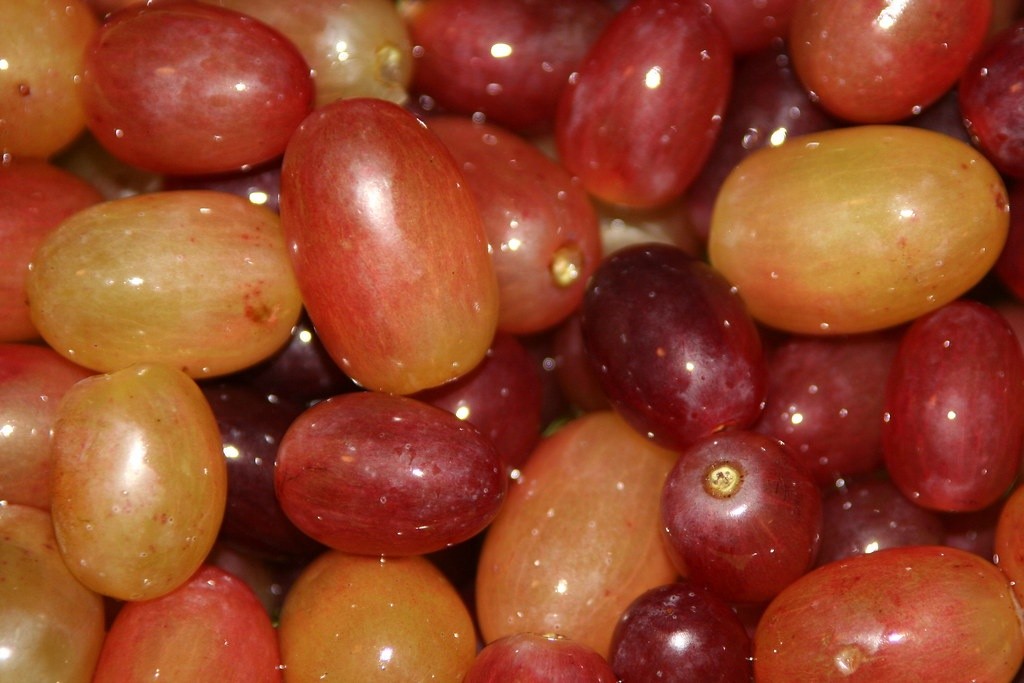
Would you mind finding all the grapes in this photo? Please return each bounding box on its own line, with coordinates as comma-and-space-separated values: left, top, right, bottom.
0, 0, 1024, 683
